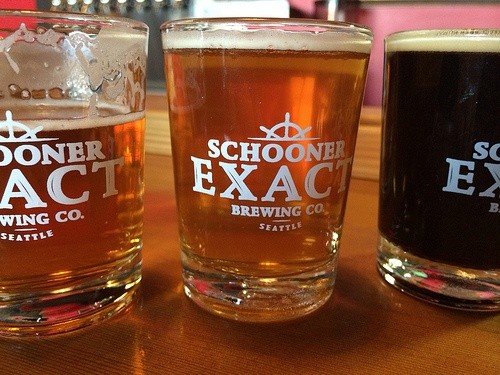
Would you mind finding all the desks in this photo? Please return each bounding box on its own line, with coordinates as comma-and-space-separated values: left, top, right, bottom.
2, 89, 500, 372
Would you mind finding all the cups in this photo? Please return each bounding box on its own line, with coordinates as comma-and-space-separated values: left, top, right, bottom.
159, 13, 372, 324
1, 6, 148, 338
378, 21, 500, 311
